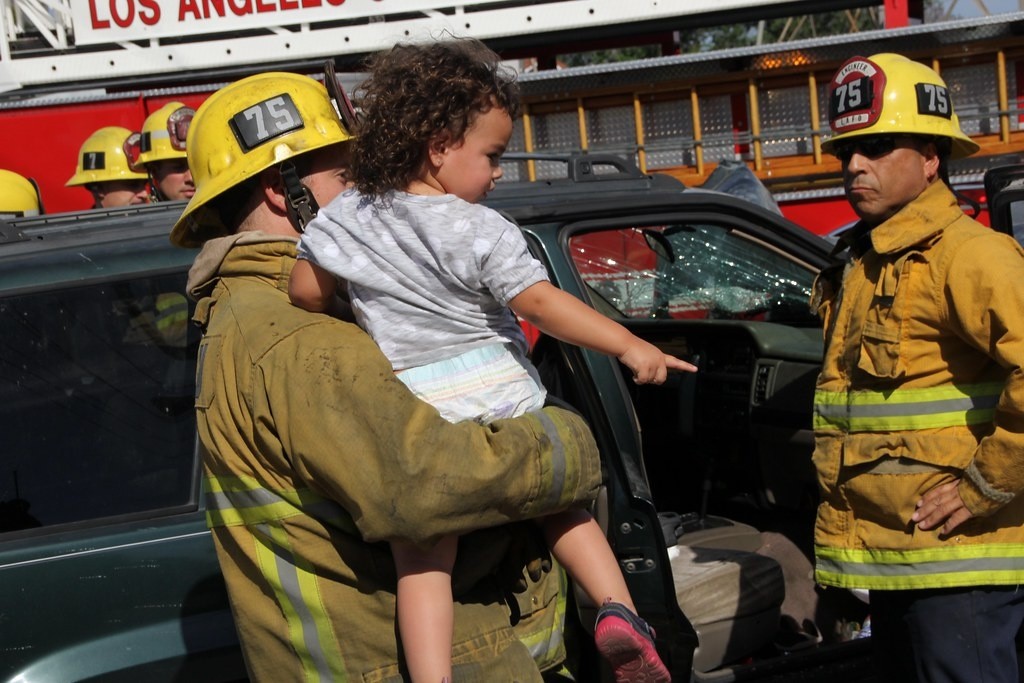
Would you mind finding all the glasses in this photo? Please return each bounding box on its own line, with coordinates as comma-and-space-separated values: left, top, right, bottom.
832, 134, 928, 163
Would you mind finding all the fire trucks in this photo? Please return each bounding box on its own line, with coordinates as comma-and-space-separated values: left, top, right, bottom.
0, 0, 1023, 329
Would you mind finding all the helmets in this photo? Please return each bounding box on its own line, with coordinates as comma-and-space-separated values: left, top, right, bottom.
169, 71, 367, 249
131, 101, 196, 173
64, 126, 153, 187
0, 169, 39, 220
820, 53, 980, 160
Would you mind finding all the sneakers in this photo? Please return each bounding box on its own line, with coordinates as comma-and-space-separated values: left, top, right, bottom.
594, 597, 671, 683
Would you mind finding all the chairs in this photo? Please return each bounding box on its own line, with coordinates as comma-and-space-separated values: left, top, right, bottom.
667, 544, 786, 672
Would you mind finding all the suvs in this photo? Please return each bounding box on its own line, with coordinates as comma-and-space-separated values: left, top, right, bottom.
1, 152, 871, 683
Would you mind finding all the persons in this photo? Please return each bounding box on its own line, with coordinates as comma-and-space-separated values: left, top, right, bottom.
288, 41, 697, 683
811, 54, 1024, 683
0, 103, 195, 350
170, 72, 603, 683
